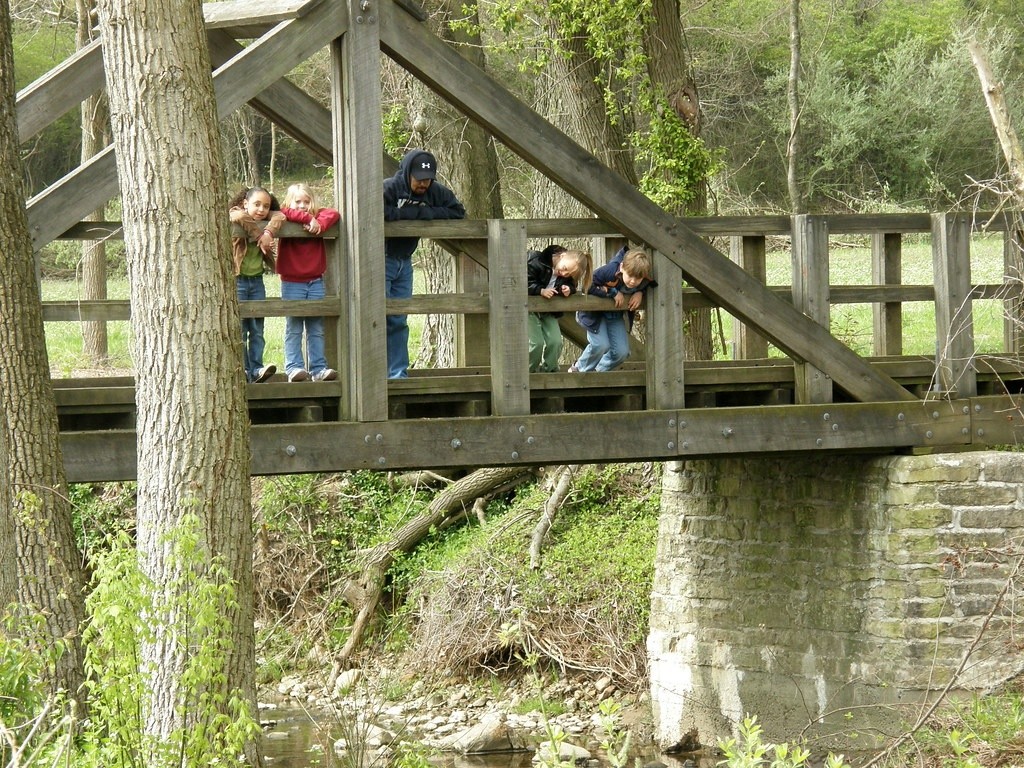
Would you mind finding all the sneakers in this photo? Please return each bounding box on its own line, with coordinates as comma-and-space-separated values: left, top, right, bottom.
410, 153, 438, 181
288, 368, 308, 382
312, 368, 338, 382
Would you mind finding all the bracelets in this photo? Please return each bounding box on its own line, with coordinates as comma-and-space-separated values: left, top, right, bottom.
265, 229, 274, 238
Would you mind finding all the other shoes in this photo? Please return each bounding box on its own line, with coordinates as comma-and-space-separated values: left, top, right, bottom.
252, 363, 277, 383
567, 361, 581, 373
245, 370, 250, 383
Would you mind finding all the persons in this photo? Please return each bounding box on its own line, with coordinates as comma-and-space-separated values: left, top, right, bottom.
282, 183, 340, 381
383, 149, 464, 377
568, 238, 658, 373
527, 244, 594, 371
228, 188, 285, 384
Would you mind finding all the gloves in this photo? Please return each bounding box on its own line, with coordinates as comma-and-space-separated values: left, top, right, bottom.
401, 205, 419, 219
419, 206, 435, 220
